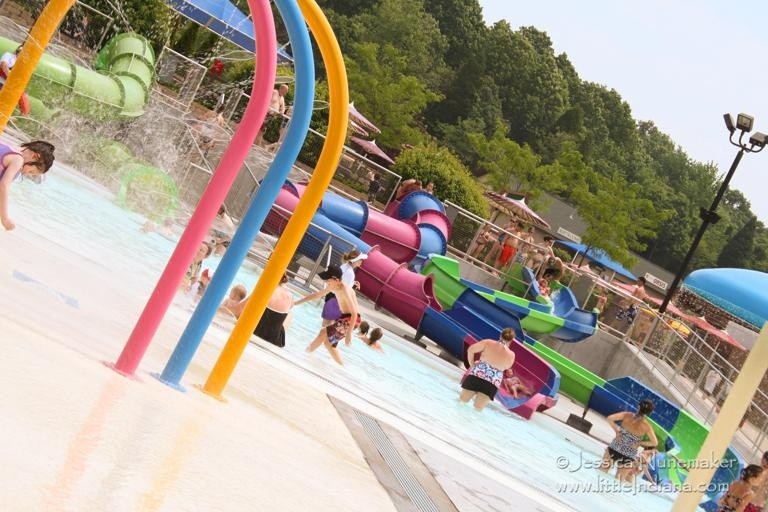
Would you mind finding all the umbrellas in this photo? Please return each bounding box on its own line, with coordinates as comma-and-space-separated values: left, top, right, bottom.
347, 119, 368, 136
482, 189, 551, 233
349, 100, 382, 134
347, 135, 396, 173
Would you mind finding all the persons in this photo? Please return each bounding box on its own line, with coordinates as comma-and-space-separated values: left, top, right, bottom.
267, 83, 289, 115
264, 106, 294, 152
0, 139, 56, 231
743, 450, 768, 512
183, 202, 383, 370
0, 44, 23, 89
367, 174, 434, 203
592, 290, 610, 315
468, 217, 564, 298
600, 399, 660, 481
598, 276, 649, 342
502, 367, 532, 400
457, 324, 518, 408
715, 463, 765, 512
625, 446, 660, 486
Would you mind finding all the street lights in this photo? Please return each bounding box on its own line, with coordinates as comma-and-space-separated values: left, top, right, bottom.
637, 112, 768, 355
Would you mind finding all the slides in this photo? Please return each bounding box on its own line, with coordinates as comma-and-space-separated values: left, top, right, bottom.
0, 31, 178, 229
251, 176, 561, 421
415, 252, 743, 512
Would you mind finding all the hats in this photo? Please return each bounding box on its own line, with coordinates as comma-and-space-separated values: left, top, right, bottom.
350, 251, 368, 263
318, 264, 343, 280
